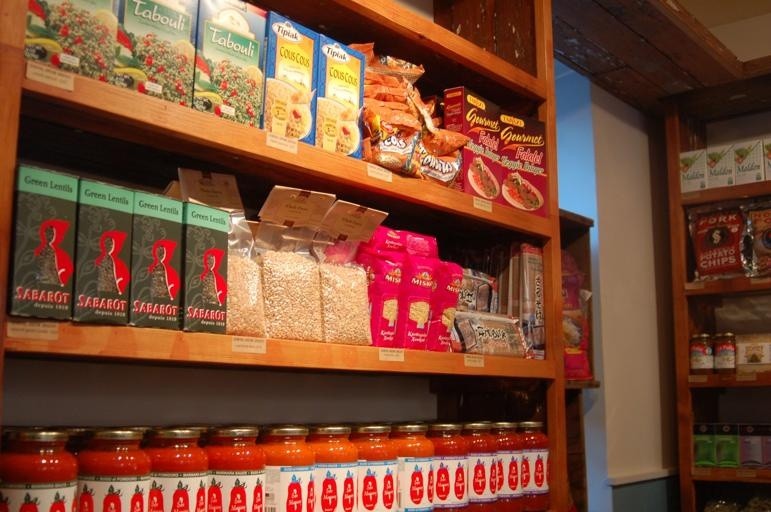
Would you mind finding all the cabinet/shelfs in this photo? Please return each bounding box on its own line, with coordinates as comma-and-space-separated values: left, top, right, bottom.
559, 208, 602, 511
0, 0, 570, 512
663, 72, 771, 511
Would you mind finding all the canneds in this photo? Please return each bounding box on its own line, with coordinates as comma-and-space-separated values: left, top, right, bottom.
0, 416, 554, 512
690, 332, 737, 376
